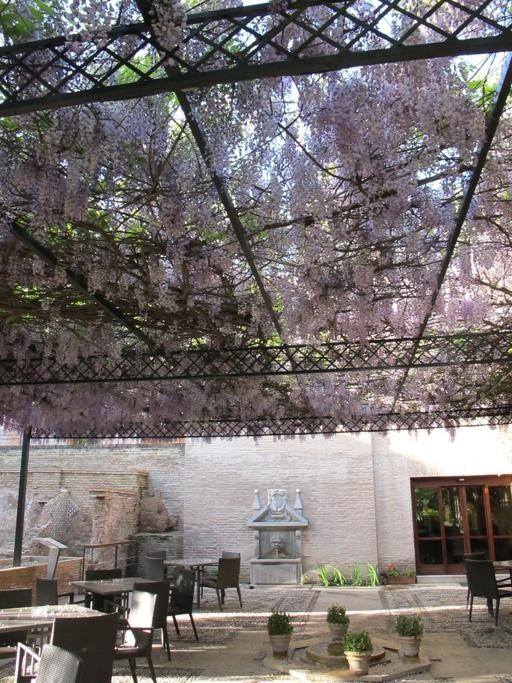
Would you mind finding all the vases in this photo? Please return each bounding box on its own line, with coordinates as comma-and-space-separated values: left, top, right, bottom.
387, 576, 416, 584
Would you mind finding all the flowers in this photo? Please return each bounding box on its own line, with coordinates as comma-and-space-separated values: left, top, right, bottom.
386, 561, 416, 576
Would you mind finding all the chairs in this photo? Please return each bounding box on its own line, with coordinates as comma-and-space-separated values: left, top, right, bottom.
0, 549, 243, 683
465, 559, 512, 626
462, 553, 512, 614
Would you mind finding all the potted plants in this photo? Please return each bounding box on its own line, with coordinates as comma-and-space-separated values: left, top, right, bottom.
343, 630, 373, 673
326, 602, 349, 636
394, 614, 424, 657
267, 611, 294, 652
